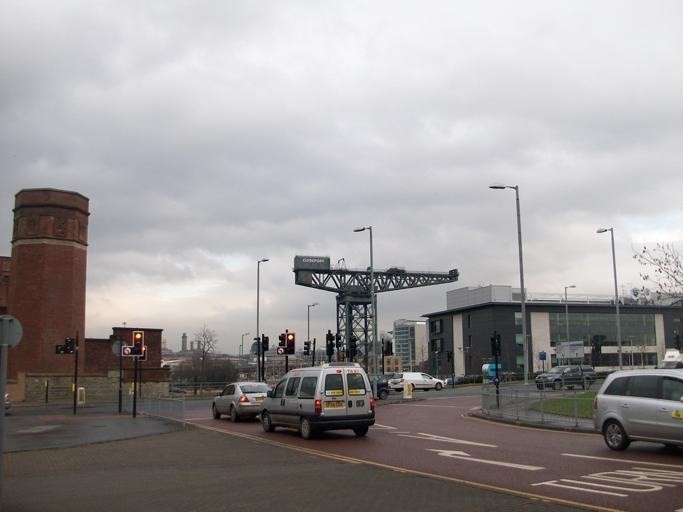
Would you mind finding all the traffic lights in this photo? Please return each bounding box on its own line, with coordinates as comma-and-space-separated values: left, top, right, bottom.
335, 333, 342, 348
325, 333, 335, 356
262, 334, 269, 353
55, 335, 74, 355
349, 336, 357, 358
138, 346, 147, 362
278, 333, 285, 346
285, 332, 295, 355
133, 331, 144, 357
302, 340, 311, 355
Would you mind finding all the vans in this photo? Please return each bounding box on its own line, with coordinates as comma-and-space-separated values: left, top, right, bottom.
593, 368, 682, 450
258, 362, 376, 440
389, 372, 447, 393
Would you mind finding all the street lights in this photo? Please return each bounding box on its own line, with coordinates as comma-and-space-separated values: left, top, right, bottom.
622, 334, 650, 369
596, 226, 623, 370
240, 334, 248, 358
256, 258, 269, 382
458, 346, 471, 384
238, 344, 242, 357
307, 302, 319, 341
352, 226, 378, 400
254, 337, 261, 382
564, 284, 576, 342
488, 184, 529, 385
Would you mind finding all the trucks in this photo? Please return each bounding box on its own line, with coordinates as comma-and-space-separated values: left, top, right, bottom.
661, 347, 683, 368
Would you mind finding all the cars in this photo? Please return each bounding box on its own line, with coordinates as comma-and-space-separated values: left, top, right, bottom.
444, 371, 518, 385
212, 381, 274, 422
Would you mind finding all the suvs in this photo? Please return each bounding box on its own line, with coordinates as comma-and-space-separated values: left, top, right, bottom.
367, 374, 390, 400
535, 364, 597, 390
